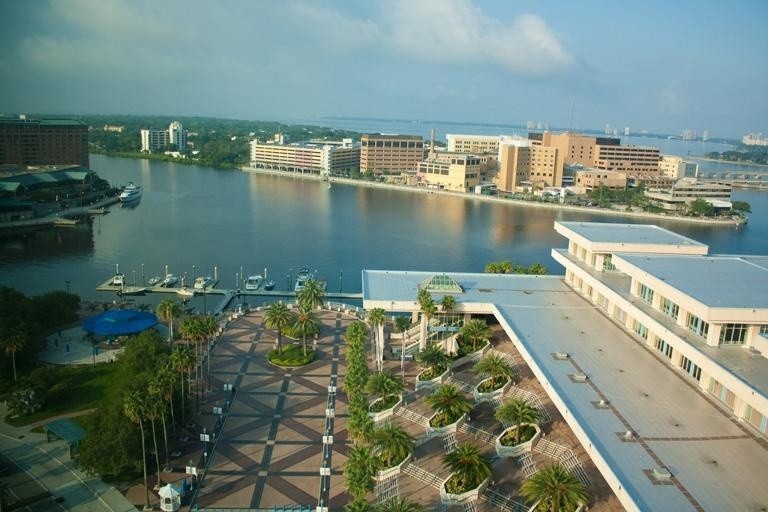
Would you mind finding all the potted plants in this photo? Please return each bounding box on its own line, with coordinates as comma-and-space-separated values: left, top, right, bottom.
369, 421, 414, 484
492, 398, 543, 460
459, 319, 492, 361
471, 352, 515, 398
414, 343, 454, 387
426, 383, 473, 434
369, 369, 404, 422
521, 462, 592, 512
440, 442, 492, 505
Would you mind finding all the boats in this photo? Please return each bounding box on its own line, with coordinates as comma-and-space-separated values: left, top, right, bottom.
163, 277, 178, 285
265, 280, 275, 291
246, 275, 262, 289
194, 276, 212, 289
295, 268, 313, 292
118, 184, 142, 202
148, 277, 160, 285
113, 273, 124, 284
